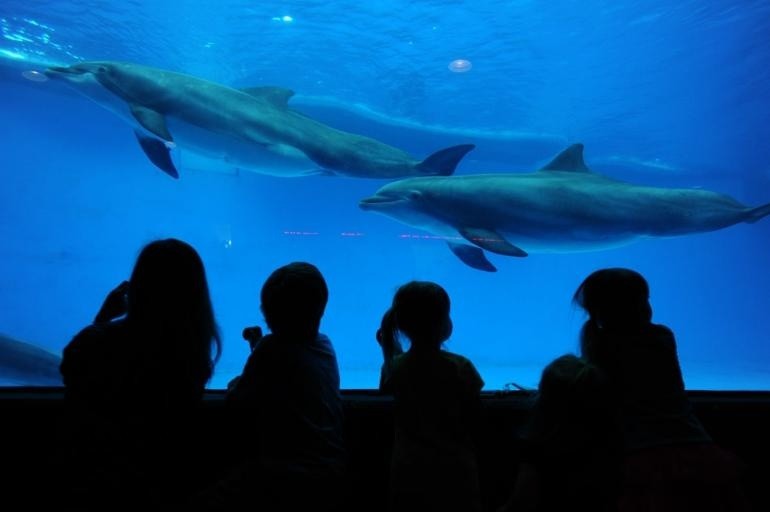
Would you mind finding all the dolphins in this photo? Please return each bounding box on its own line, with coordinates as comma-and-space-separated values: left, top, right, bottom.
358, 144, 770, 273
43, 61, 476, 179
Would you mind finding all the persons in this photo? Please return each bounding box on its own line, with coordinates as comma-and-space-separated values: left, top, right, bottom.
225, 263, 346, 479
377, 281, 482, 444
60, 238, 220, 454
539, 268, 686, 512
